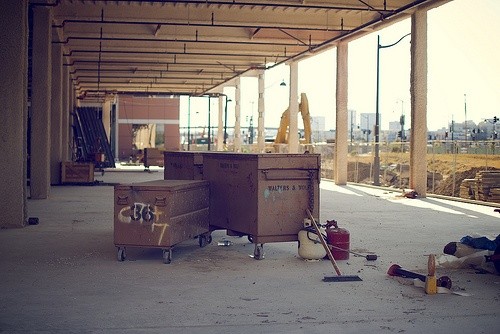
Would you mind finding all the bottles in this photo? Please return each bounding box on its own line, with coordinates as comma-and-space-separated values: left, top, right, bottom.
217, 240, 235, 246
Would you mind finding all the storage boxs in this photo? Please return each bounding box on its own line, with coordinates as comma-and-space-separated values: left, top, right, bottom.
143, 147, 165, 168
202, 152, 322, 244
60, 161, 96, 184
162, 150, 204, 181
114, 179, 211, 248
